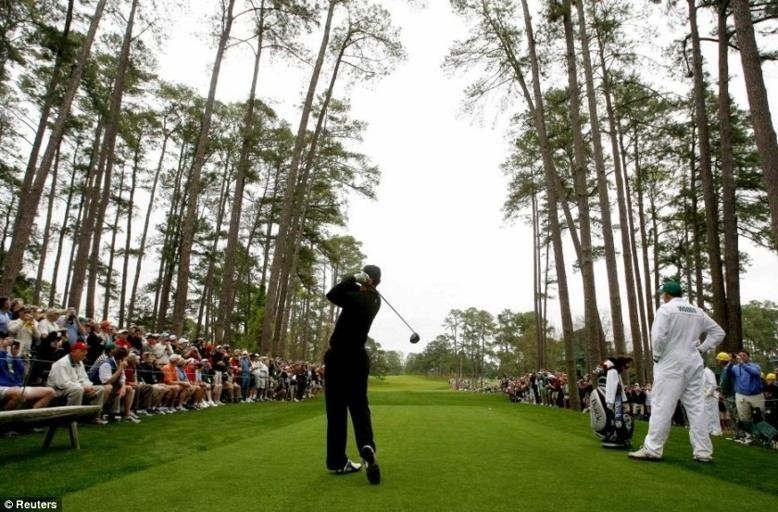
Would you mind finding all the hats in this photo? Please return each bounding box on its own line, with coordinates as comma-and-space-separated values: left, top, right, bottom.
13, 303, 260, 363
656, 281, 682, 295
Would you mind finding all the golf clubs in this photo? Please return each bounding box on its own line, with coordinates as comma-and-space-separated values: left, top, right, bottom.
583, 360, 614, 389
369, 284, 420, 344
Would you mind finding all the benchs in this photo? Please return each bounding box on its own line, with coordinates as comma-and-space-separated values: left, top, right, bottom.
0, 402, 107, 455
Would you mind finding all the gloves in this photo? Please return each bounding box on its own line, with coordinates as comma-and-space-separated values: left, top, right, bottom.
354, 271, 370, 283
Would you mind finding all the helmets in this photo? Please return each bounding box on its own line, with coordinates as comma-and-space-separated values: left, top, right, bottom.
765, 372, 776, 380
715, 351, 730, 361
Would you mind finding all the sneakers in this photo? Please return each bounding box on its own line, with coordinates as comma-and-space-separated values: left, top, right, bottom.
324, 460, 362, 474
91, 395, 305, 425
627, 448, 661, 461
360, 444, 380, 485
690, 454, 712, 463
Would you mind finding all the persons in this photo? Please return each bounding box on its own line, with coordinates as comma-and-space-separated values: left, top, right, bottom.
323, 264, 381, 485
448, 351, 777, 449
629, 280, 726, 462
0, 296, 325, 437
